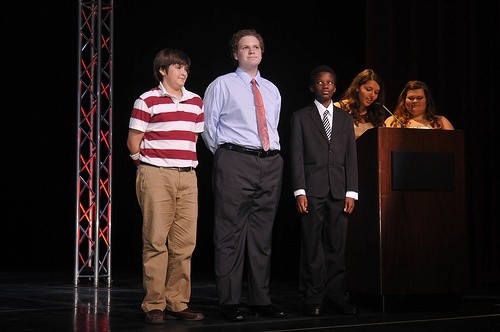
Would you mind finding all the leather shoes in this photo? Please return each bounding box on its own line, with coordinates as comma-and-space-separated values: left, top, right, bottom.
145, 310, 165, 323
305, 305, 321, 317
334, 304, 358, 315
222, 306, 244, 321
167, 310, 204, 321
253, 305, 285, 319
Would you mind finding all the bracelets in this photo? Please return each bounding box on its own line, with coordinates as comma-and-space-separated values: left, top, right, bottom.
130, 152, 140, 161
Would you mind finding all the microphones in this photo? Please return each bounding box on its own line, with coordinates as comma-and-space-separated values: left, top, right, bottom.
375, 102, 404, 128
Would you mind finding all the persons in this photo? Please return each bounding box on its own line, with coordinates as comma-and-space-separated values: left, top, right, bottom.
383, 81, 455, 130
127, 48, 204, 323
288, 66, 359, 316
200, 29, 283, 322
334, 69, 387, 313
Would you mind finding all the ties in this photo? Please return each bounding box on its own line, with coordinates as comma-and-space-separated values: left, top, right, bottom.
323, 110, 331, 141
251, 79, 269, 151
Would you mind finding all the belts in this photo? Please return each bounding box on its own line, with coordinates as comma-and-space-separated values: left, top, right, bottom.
220, 144, 279, 158
139, 160, 194, 173
